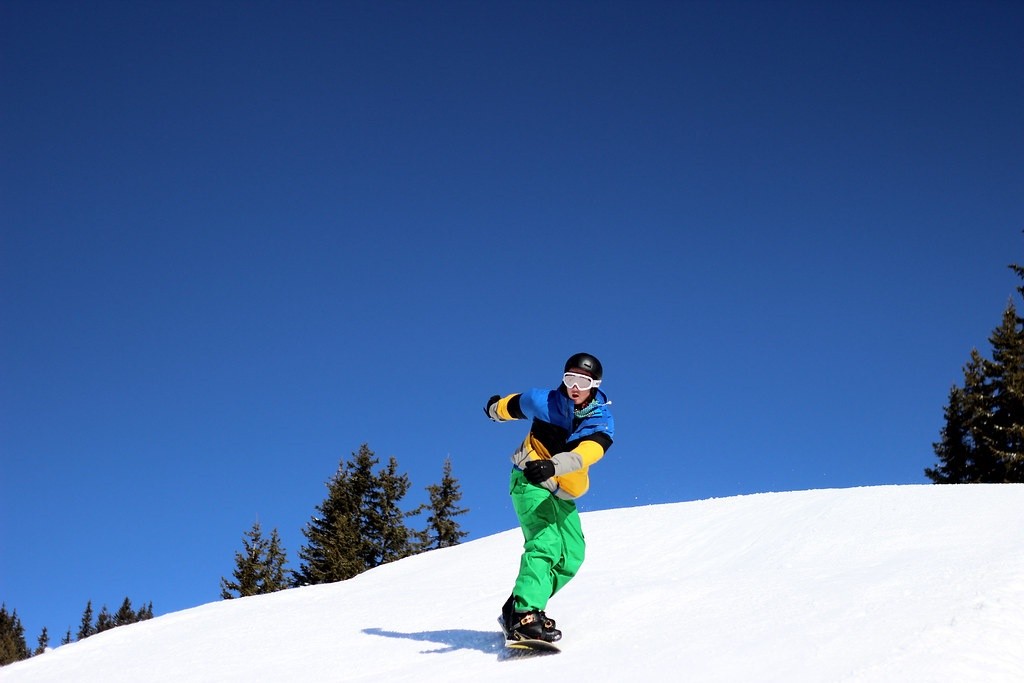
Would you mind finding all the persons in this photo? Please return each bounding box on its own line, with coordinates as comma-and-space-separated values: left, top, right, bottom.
482, 353, 616, 643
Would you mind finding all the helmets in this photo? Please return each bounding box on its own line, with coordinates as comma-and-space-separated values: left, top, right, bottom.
565, 353, 602, 378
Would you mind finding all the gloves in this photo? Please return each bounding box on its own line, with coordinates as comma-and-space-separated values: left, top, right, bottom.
483, 395, 500, 422
523, 459, 554, 484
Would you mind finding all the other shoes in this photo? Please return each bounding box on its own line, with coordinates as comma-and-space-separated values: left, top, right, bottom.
509, 609, 563, 641
502, 598, 556, 628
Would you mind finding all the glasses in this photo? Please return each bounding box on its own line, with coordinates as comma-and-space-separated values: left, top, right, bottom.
562, 372, 602, 390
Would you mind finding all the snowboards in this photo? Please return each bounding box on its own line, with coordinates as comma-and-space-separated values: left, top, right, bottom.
496, 614, 562, 653
